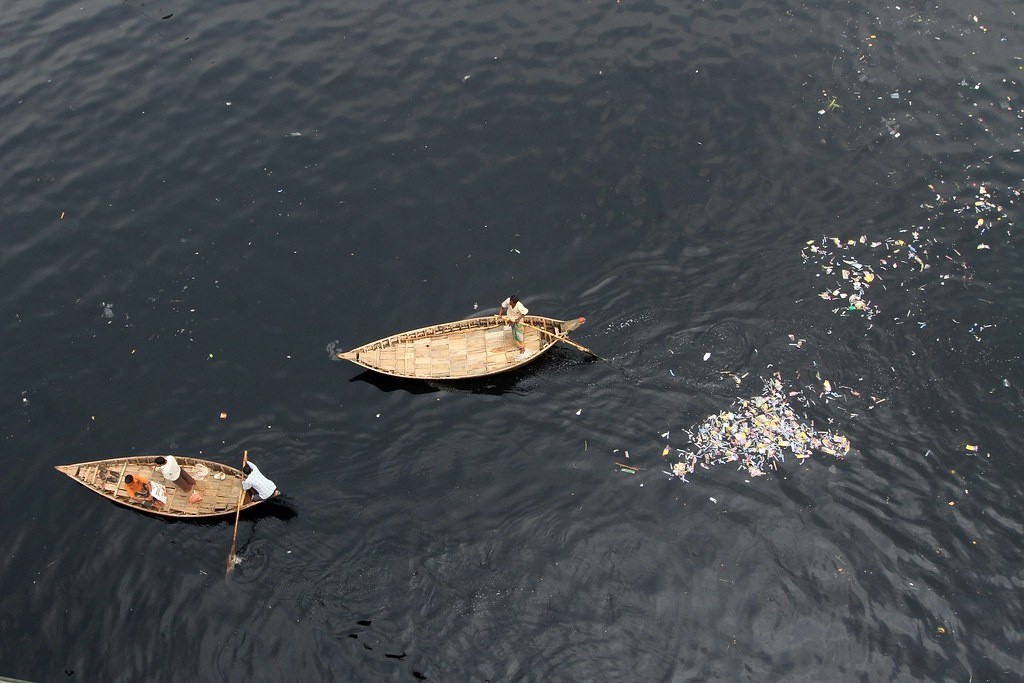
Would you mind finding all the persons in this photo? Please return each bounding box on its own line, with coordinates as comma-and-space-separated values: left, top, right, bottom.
241, 458, 277, 501
124, 475, 164, 509
498, 295, 529, 353
156, 455, 195, 491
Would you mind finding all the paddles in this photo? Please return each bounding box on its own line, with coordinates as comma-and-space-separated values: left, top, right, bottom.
493, 313, 599, 358
230, 449, 248, 560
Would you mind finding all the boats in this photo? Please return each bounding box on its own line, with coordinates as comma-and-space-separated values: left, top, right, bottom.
54, 455, 281, 517
336, 315, 586, 380
